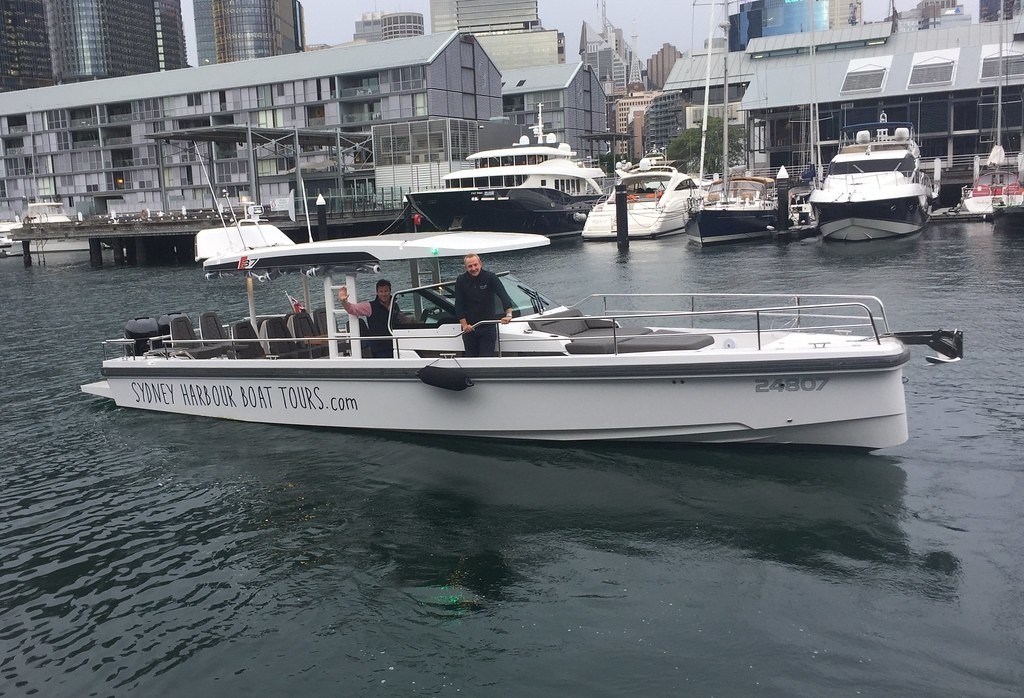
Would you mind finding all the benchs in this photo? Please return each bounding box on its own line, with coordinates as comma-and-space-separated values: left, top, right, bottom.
528, 308, 716, 354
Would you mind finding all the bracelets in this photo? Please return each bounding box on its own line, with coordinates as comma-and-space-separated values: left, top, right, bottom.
505, 312, 512, 317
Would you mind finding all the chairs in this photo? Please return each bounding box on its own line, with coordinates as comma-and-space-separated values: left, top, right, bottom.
171, 307, 374, 360
972, 184, 990, 196
708, 193, 720, 205
1006, 182, 1020, 195
645, 193, 655, 198
740, 191, 753, 204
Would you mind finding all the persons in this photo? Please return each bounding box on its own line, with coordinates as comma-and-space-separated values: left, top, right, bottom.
339, 279, 419, 358
454, 252, 512, 357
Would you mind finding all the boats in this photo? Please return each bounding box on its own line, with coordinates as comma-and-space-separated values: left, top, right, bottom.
405, 101, 612, 241
959, 1, 1024, 215
78, 256, 965, 454
192, 215, 557, 280
807, 120, 934, 241
579, 152, 709, 242
680, 174, 792, 249
